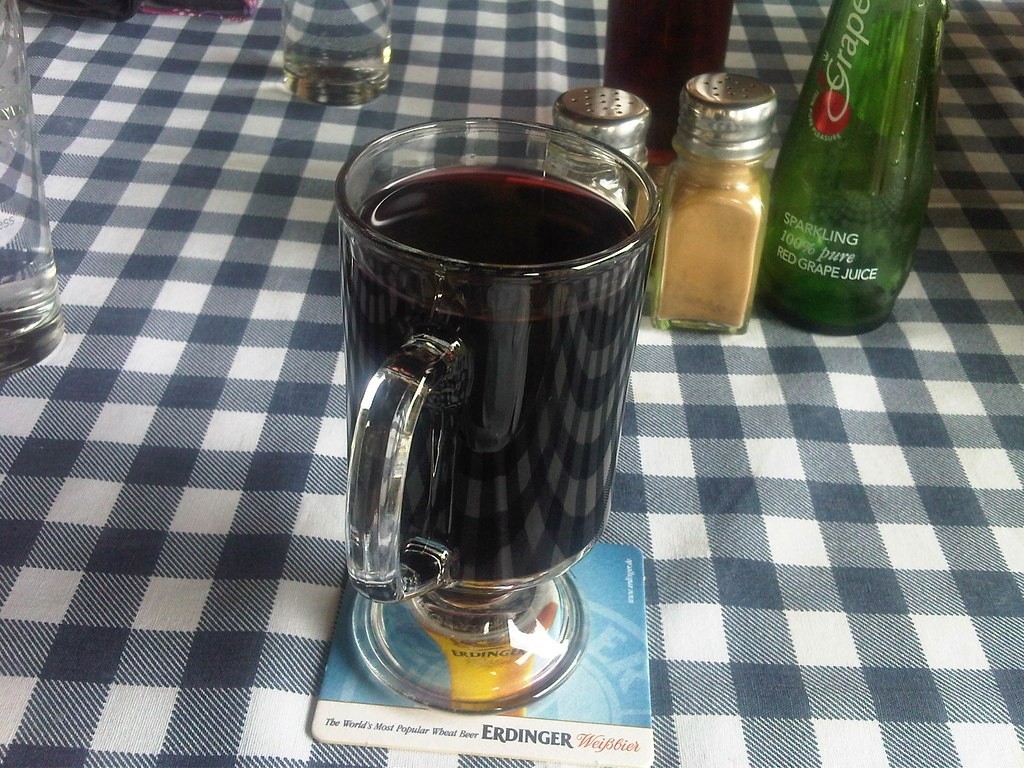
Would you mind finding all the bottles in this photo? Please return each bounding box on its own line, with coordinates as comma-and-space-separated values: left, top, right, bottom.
542, 86, 654, 233
752, 0, 949, 335
652, 72, 781, 337
602, 0, 735, 169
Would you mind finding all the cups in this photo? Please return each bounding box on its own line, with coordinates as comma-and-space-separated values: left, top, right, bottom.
335, 115, 661, 716
0, 0, 65, 378
280, 0, 391, 107
